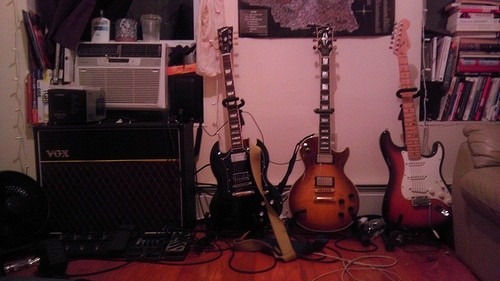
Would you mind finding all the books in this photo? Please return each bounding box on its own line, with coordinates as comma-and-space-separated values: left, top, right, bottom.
22, 8, 76, 126
427, 0, 500, 122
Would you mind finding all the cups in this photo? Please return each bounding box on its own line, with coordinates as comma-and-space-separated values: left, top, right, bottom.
140, 14, 161, 43
114, 18, 138, 42
91, 18, 110, 42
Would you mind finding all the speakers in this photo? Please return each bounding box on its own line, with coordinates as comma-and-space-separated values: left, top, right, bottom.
46, 87, 106, 123
74, 41, 167, 110
37, 120, 196, 234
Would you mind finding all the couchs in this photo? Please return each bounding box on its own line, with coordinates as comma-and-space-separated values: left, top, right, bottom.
450, 124, 500, 281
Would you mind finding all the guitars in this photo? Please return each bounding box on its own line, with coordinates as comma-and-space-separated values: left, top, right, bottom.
288, 25, 360, 233
209, 25, 282, 230
379, 18, 453, 233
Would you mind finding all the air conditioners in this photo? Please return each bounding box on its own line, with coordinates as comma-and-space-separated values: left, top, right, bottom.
74, 42, 169, 111
